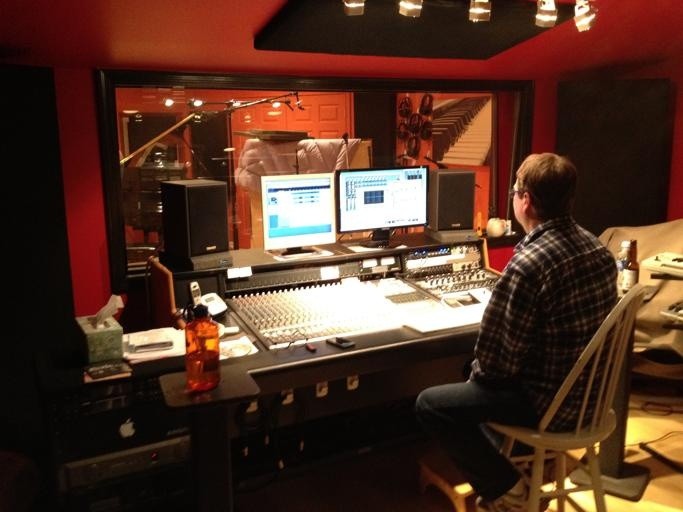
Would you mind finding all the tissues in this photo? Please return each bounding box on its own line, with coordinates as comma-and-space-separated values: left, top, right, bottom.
71, 294, 125, 369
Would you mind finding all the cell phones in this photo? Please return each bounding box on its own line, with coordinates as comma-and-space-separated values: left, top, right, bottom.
189, 281, 201, 306
325, 336, 355, 349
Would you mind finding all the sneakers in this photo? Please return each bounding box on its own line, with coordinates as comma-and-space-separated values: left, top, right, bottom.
544, 454, 578, 484
477, 483, 548, 511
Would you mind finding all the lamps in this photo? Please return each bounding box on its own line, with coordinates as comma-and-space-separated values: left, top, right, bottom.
398, 0, 423, 17
574, 0, 600, 32
187, 98, 203, 107
469, 0, 493, 22
267, 99, 282, 108
343, 0, 365, 16
534, 0, 559, 29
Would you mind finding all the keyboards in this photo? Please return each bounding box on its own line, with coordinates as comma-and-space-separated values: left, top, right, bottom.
659, 301, 682, 319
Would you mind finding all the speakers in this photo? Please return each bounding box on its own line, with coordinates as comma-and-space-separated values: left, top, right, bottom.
423, 168, 479, 242
160, 179, 228, 257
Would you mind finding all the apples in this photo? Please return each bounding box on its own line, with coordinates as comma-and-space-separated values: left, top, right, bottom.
486, 217, 506, 238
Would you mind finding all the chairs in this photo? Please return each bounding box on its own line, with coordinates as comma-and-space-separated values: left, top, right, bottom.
481, 282, 648, 511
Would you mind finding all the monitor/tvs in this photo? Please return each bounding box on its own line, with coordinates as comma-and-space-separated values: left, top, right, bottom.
260, 173, 336, 258
335, 165, 429, 248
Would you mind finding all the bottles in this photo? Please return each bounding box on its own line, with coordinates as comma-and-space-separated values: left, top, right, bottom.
184, 304, 220, 392
622, 239, 639, 294
616, 241, 630, 290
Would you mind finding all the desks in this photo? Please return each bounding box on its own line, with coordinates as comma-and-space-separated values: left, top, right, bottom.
140, 237, 613, 512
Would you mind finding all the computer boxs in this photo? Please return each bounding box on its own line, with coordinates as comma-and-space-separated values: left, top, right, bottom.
54, 399, 198, 512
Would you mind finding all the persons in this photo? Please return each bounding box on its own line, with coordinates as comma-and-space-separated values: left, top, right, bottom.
414, 152, 620, 511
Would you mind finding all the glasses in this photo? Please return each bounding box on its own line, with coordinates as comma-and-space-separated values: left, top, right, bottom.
508, 188, 521, 198
